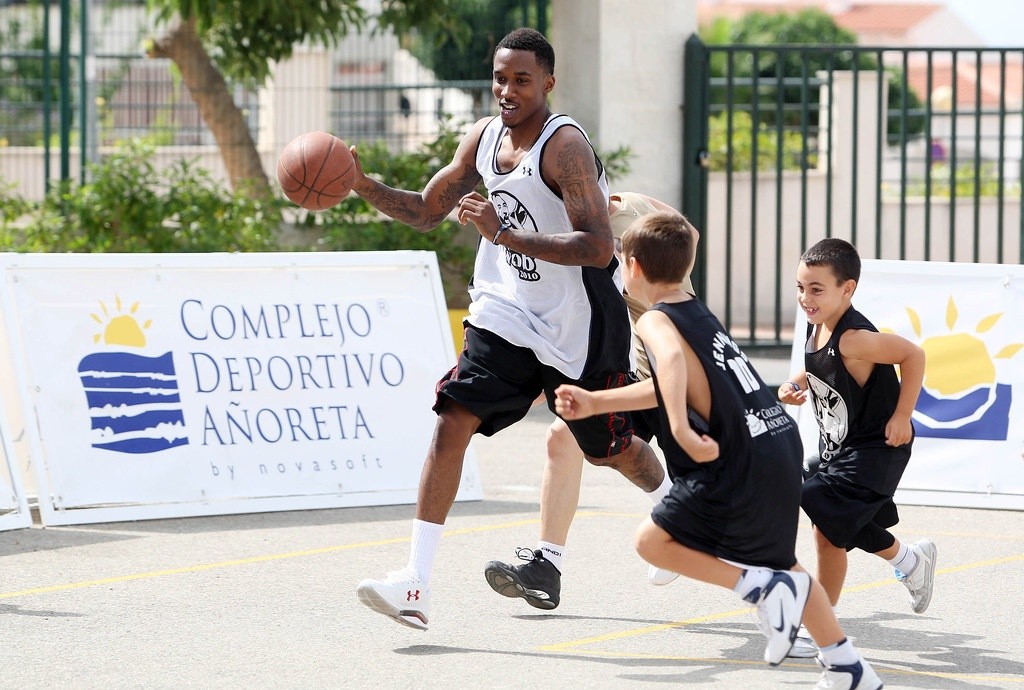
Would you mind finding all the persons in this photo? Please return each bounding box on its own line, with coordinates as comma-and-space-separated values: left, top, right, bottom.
396, 87, 413, 139
777, 239, 938, 659
348, 26, 682, 633
553, 208, 884, 690
484, 171, 702, 615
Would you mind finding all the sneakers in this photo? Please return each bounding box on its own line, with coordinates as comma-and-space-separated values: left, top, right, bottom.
485, 547, 561, 610
811, 656, 883, 690
740, 570, 811, 666
647, 565, 679, 586
356, 568, 429, 630
895, 542, 937, 614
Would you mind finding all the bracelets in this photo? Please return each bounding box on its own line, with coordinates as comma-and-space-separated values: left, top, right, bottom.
491, 218, 513, 248
785, 379, 802, 393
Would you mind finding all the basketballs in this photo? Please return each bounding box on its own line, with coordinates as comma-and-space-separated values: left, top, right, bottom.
278, 131, 356, 211
